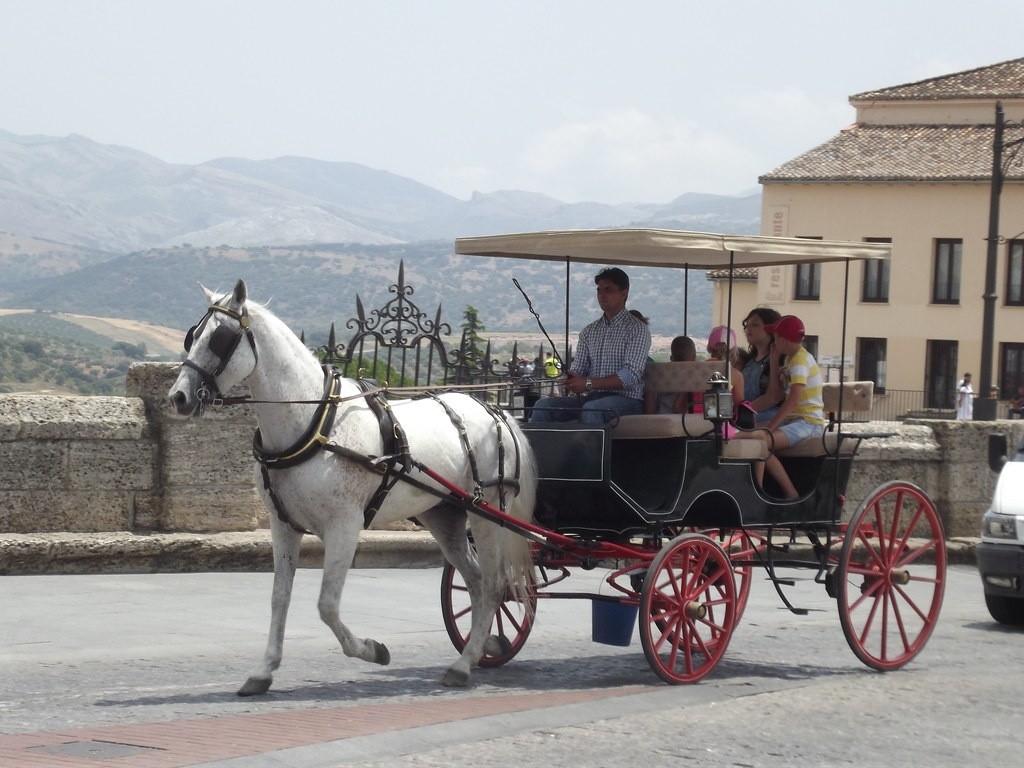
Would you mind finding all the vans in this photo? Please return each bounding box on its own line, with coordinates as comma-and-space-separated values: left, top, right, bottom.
977, 430, 1024, 628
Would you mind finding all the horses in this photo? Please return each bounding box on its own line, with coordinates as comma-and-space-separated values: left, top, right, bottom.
164, 280, 541, 698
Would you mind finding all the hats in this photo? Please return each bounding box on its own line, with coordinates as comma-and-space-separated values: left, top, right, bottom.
708, 326, 736, 351
763, 315, 805, 342
991, 385, 1000, 391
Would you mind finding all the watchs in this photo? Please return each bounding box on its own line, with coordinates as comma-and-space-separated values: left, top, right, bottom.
586, 379, 592, 391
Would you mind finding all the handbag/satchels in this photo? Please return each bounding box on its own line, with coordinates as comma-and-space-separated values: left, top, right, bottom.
733, 400, 758, 430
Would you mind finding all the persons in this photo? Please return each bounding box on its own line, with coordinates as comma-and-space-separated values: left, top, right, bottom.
522, 358, 538, 374
531, 267, 652, 423
739, 308, 787, 424
751, 315, 824, 501
655, 336, 696, 414
692, 325, 766, 489
629, 310, 654, 362
956, 373, 973, 421
1008, 387, 1024, 420
544, 352, 561, 394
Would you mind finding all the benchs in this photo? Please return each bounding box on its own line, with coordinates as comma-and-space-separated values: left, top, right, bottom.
769, 380, 875, 517
564, 363, 768, 509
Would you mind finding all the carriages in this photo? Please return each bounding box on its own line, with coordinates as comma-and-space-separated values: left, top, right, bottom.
162, 227, 949, 697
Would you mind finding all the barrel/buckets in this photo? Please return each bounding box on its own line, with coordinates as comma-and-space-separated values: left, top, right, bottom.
591, 598, 639, 648
